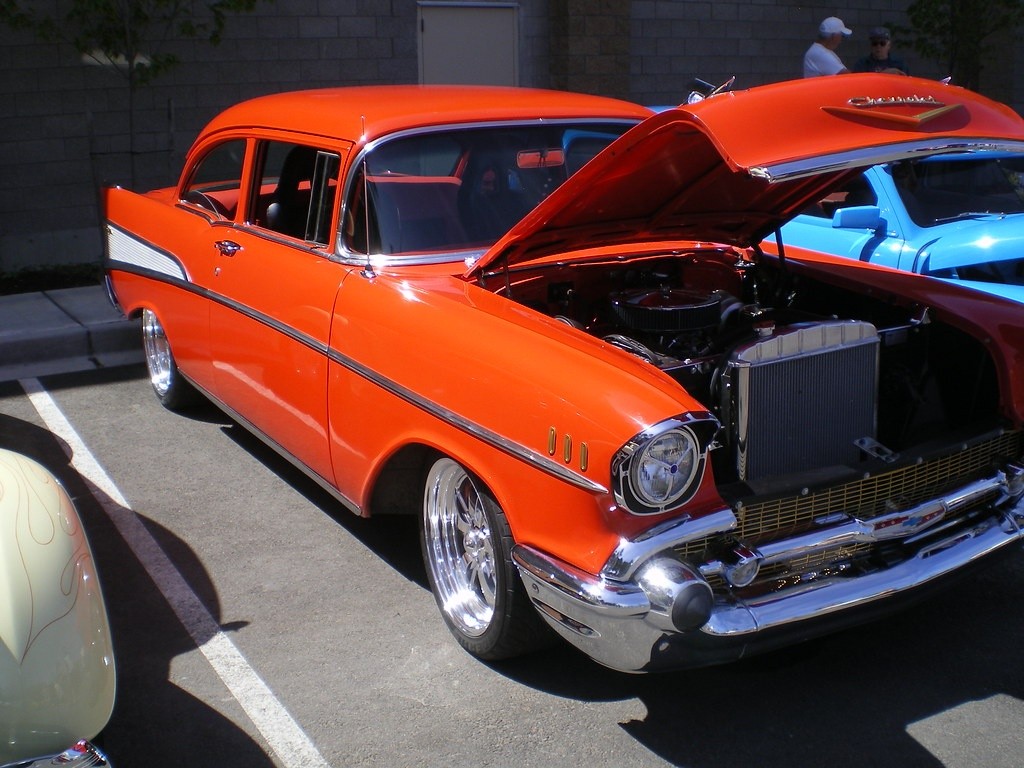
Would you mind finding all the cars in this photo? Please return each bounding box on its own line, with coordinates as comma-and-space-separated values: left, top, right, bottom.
647, 77, 1024, 302
104, 69, 1023, 674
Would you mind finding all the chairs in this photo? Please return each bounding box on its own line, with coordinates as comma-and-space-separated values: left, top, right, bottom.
265, 141, 559, 251
